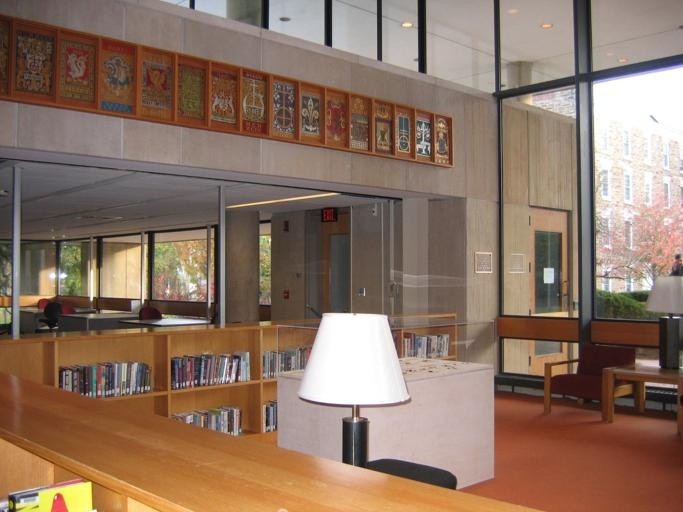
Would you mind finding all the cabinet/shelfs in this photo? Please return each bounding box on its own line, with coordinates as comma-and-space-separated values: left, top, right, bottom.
2, 312, 458, 444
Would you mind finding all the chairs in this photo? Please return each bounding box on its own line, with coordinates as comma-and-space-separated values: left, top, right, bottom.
210, 312, 219, 323
139, 306, 163, 319
38, 303, 63, 329
61, 299, 75, 313
543, 342, 638, 421
367, 460, 458, 492
39, 298, 51, 309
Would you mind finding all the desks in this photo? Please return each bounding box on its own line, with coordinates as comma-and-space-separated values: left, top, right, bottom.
20, 306, 44, 332
608, 364, 683, 432
277, 353, 495, 492
119, 314, 211, 327
58, 311, 140, 329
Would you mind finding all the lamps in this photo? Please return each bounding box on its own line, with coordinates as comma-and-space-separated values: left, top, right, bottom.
299, 313, 412, 468
644, 277, 683, 371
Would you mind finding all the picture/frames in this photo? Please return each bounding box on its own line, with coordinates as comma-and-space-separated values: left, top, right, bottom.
0, 12, 454, 169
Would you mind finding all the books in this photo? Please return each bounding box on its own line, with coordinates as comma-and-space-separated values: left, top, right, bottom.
59, 360, 151, 398
404, 334, 448, 359
170, 351, 251, 390
173, 405, 242, 435
263, 400, 278, 433
263, 344, 312, 379
0, 479, 92, 512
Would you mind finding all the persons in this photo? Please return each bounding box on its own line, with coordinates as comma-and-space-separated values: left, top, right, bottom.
671, 252, 683, 275
